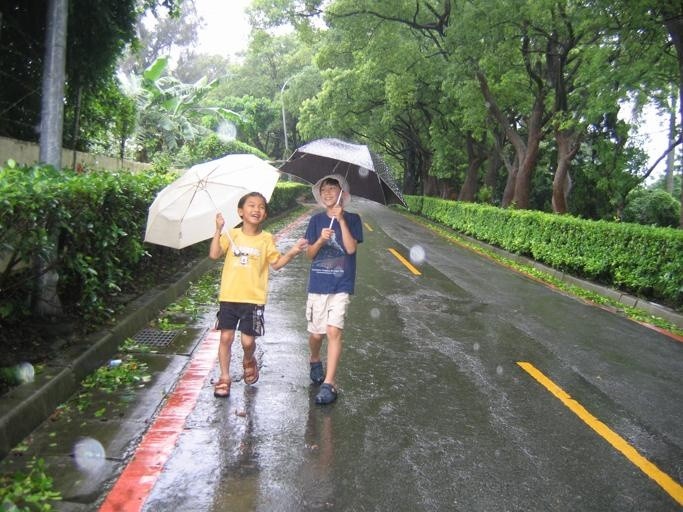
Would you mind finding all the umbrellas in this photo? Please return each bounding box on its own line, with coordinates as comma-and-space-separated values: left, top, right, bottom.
143, 154, 281, 257
280, 138, 410, 214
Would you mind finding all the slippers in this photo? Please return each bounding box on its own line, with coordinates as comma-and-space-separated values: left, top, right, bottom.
310, 361, 325, 384
315, 383, 337, 404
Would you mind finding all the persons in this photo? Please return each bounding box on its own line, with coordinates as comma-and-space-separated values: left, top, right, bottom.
209, 192, 308, 397
302, 174, 363, 403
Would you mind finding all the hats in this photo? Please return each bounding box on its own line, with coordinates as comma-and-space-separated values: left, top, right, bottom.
312, 173, 352, 209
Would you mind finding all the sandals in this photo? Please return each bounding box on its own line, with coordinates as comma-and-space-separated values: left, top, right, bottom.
243, 356, 259, 384
214, 379, 231, 397
